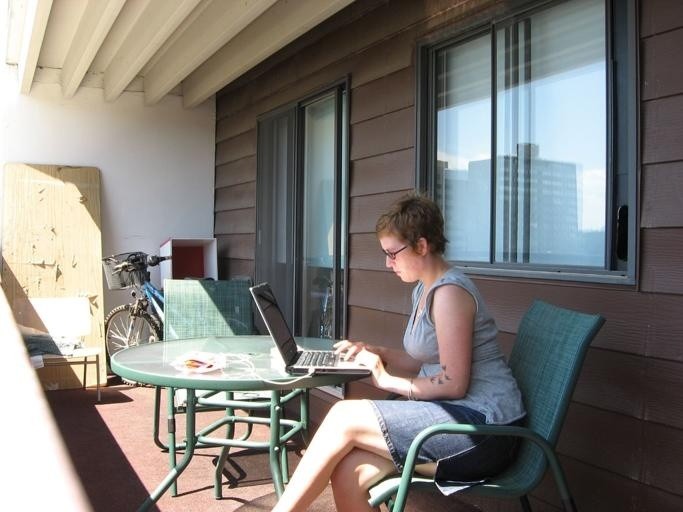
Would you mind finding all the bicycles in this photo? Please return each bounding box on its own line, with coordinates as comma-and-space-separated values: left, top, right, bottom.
101, 251, 251, 387
303, 253, 332, 338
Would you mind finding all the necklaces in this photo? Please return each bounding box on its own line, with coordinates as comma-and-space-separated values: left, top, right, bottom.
413, 262, 453, 321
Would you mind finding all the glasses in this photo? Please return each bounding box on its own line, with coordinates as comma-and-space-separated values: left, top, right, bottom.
380, 244, 409, 260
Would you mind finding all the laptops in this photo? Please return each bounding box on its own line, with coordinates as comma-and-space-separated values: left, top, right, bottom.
247, 280, 372, 378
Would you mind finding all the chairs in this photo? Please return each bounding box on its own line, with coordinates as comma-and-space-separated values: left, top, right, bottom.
367, 299, 606, 512
154, 280, 258, 453
12, 296, 102, 401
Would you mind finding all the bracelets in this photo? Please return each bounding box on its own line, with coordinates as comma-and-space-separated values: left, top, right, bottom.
406, 375, 417, 401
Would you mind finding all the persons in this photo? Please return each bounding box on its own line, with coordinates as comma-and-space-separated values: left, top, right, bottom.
268, 188, 528, 512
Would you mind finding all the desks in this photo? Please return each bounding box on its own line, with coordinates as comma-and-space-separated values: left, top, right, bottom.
109, 336, 385, 511
